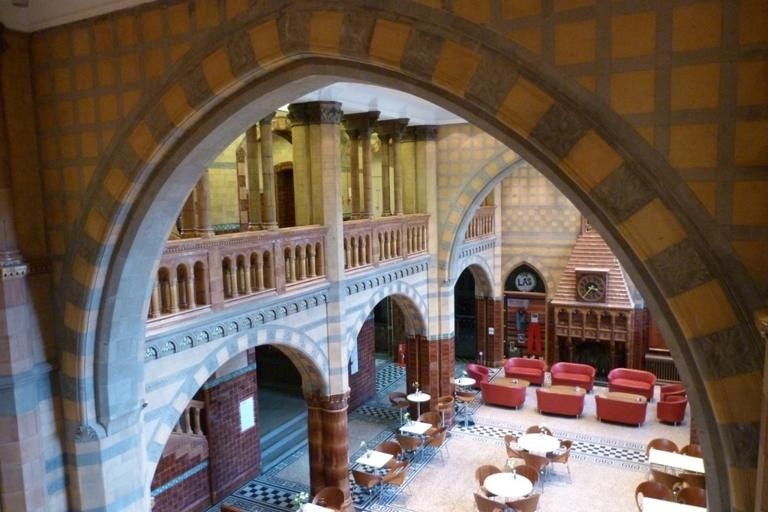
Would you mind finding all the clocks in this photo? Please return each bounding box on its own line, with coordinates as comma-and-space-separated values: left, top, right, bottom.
578, 274, 605, 301
